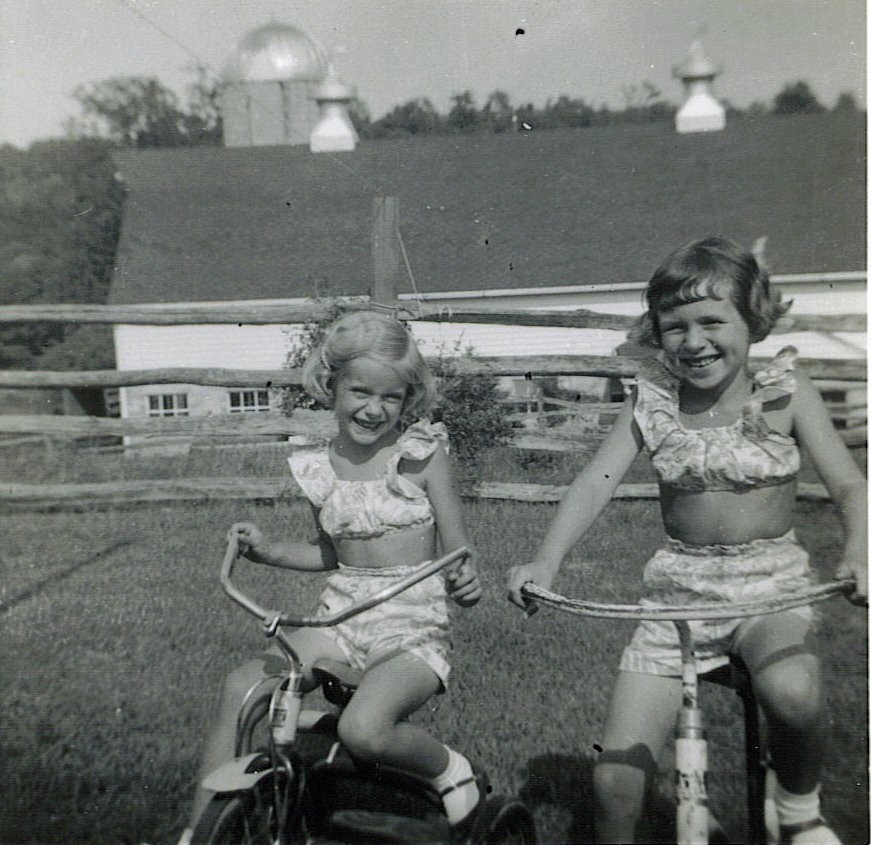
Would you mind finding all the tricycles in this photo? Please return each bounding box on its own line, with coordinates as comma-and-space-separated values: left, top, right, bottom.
162, 529, 542, 843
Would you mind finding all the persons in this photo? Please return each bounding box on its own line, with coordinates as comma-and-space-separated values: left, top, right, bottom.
179, 309, 481, 845
506, 238, 869, 845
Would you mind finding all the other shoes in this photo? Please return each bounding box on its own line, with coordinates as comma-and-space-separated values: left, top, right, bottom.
443, 753, 496, 845
782, 817, 848, 845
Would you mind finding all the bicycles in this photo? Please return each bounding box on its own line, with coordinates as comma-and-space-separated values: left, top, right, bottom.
512, 573, 865, 845
